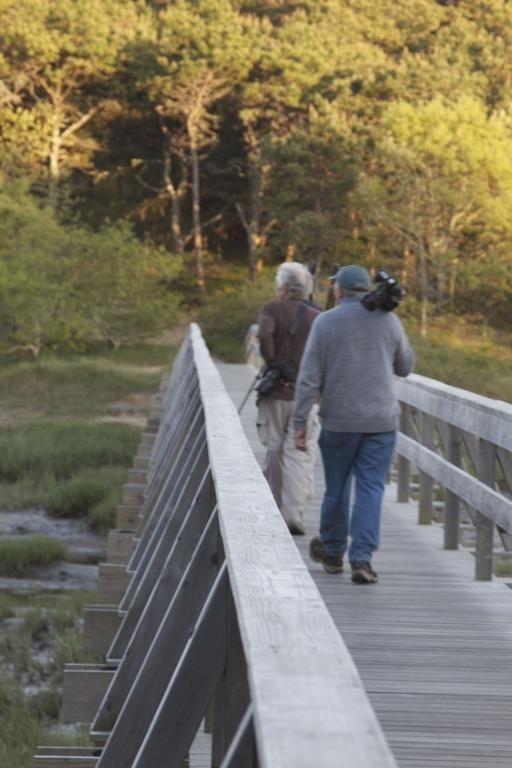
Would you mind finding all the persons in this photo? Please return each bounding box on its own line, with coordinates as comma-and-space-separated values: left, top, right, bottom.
253, 257, 323, 538
291, 262, 418, 588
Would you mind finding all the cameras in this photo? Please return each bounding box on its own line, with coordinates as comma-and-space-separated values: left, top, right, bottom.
255, 360, 295, 394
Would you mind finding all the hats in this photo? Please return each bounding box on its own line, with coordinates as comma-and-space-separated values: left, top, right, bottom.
329, 264, 373, 293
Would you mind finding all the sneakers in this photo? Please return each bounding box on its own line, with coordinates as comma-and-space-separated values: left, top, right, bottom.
287, 520, 305, 538
349, 559, 379, 585
307, 534, 345, 576
